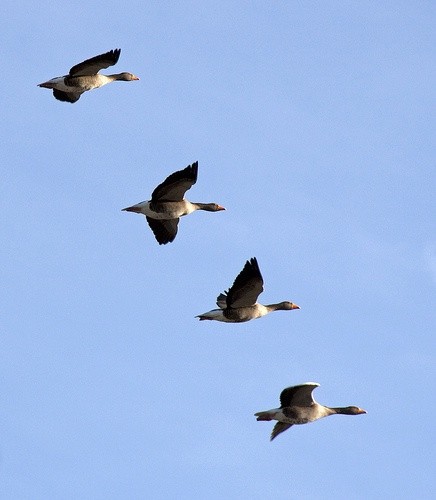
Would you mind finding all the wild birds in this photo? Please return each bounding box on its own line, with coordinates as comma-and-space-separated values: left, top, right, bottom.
254, 381, 369, 442
194, 256, 301, 323
119, 161, 227, 245
37, 48, 141, 104
